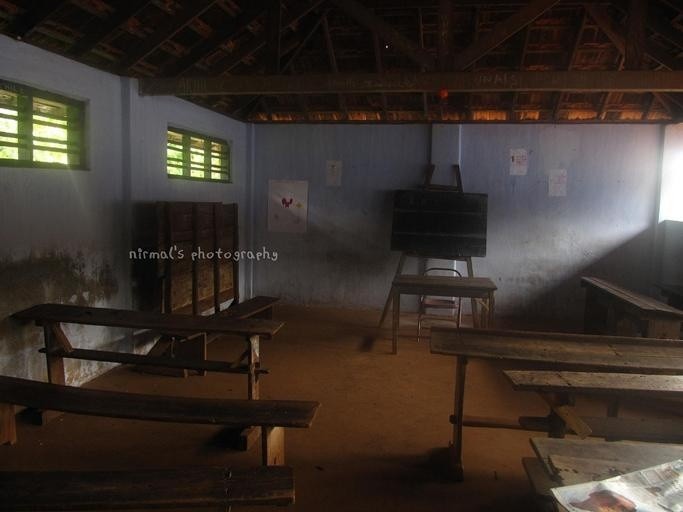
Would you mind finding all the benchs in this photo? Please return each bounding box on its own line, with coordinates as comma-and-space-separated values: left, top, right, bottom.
7, 304, 287, 399
502, 370, 683, 440
160, 296, 279, 363
429, 326, 683, 483
580, 274, 682, 338
653, 283, 682, 305
0, 374, 323, 465
0, 461, 296, 512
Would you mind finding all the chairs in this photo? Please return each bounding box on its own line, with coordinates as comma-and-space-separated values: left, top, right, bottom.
415, 268, 462, 343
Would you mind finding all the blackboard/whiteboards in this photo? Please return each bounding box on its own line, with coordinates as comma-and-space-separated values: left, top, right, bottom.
390, 189, 488, 257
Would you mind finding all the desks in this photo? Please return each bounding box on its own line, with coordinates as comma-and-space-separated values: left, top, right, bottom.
522, 438, 683, 512
392, 275, 497, 357
